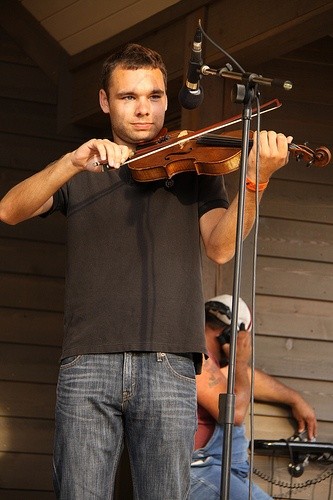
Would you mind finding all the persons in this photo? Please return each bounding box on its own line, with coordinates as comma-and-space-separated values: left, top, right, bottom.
188, 294, 318, 500
1, 44, 294, 500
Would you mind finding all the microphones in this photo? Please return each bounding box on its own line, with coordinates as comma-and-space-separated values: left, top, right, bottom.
179, 29, 204, 110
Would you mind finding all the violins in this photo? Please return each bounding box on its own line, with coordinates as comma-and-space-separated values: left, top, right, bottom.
127, 128, 331, 183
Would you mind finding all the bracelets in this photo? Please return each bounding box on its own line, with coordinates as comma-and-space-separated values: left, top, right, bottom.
246, 178, 269, 192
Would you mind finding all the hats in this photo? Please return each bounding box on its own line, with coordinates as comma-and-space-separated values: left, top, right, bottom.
205, 294, 253, 334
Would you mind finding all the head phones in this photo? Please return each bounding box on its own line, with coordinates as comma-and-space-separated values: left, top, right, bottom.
204, 301, 245, 345
